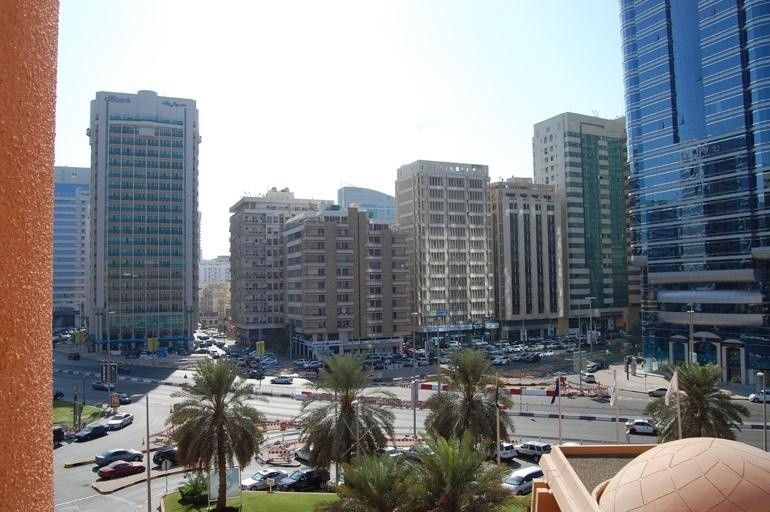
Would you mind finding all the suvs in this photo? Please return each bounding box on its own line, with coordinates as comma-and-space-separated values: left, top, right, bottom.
68, 352, 80, 361
513, 440, 553, 462
487, 442, 517, 462
278, 468, 331, 494
152, 445, 199, 467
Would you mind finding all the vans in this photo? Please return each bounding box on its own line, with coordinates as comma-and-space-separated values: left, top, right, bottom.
271, 375, 294, 384
501, 466, 543, 499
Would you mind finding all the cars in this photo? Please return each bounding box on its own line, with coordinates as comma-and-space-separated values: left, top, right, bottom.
117, 363, 131, 375
241, 468, 288, 491
107, 413, 135, 430
293, 447, 316, 461
75, 422, 109, 442
242, 369, 263, 379
52, 423, 63, 447
468, 481, 491, 501
99, 459, 145, 485
94, 447, 144, 467
292, 332, 600, 372
648, 387, 668, 397
121, 347, 189, 360
623, 418, 665, 437
580, 356, 609, 383
750, 387, 770, 405
386, 448, 434, 466
52, 327, 85, 349
326, 472, 345, 492
93, 381, 116, 391
116, 392, 130, 405
194, 329, 279, 369
627, 354, 645, 364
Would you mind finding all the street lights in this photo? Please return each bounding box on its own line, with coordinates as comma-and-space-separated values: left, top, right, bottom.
757, 372, 768, 452
578, 297, 592, 393
131, 392, 151, 511
687, 309, 692, 367
348, 398, 361, 457
412, 311, 424, 382
686, 303, 695, 367
436, 316, 442, 400
152, 322, 157, 364
585, 296, 596, 357
